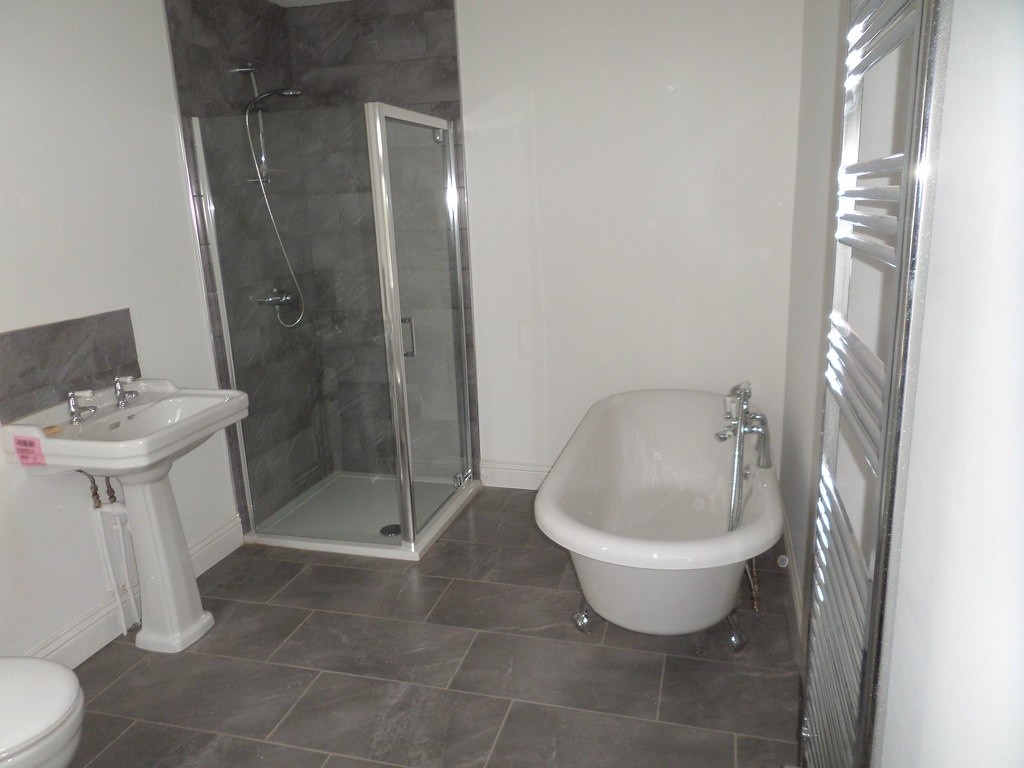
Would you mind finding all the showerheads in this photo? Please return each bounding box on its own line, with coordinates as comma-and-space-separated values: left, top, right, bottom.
248, 86, 304, 111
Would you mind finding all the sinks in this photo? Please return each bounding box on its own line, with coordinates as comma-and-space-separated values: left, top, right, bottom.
0, 378, 252, 483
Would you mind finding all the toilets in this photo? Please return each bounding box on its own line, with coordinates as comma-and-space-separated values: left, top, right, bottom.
0, 654, 87, 768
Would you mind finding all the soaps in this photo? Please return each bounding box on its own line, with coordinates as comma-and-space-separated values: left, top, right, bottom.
42, 424, 62, 438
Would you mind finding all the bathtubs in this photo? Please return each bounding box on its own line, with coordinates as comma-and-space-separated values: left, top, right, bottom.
533, 386, 787, 641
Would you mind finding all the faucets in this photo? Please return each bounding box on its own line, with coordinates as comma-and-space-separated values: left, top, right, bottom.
713, 394, 762, 443
111, 376, 141, 409
65, 389, 99, 425
